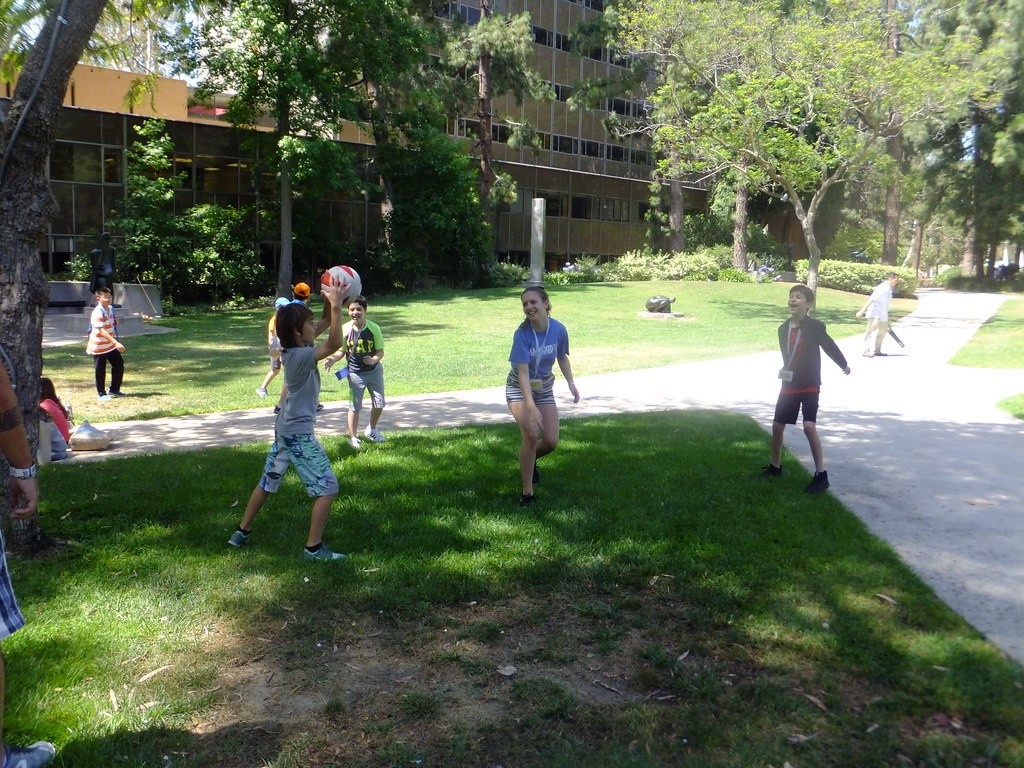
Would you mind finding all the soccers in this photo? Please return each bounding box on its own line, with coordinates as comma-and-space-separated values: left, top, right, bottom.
321, 265, 363, 308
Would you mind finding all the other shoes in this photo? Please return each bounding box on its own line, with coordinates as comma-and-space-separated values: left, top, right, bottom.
97, 394, 111, 400
107, 389, 126, 397
316, 404, 323, 410
255, 386, 268, 397
273, 405, 280, 415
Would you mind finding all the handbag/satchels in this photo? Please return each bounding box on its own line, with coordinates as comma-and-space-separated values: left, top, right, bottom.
35, 414, 120, 466
88, 276, 96, 292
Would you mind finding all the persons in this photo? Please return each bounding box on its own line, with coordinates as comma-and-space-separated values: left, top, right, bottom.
856, 273, 898, 357
87, 287, 126, 400
274, 282, 323, 413
40, 378, 69, 461
256, 297, 290, 397
506, 286, 580, 509
91, 233, 115, 304
228, 274, 351, 565
760, 285, 850, 494
0, 360, 56, 768
324, 295, 386, 447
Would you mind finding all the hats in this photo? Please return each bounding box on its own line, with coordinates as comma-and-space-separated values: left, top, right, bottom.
274, 296, 291, 310
294, 283, 310, 298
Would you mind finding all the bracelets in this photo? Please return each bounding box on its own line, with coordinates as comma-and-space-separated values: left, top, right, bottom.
8, 461, 36, 479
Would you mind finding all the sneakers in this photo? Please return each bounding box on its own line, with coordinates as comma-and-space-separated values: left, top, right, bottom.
303, 545, 349, 564
760, 465, 782, 477
365, 425, 387, 443
518, 491, 537, 509
229, 530, 248, 547
4, 741, 55, 768
351, 437, 362, 448
532, 461, 540, 485
803, 471, 829, 495
